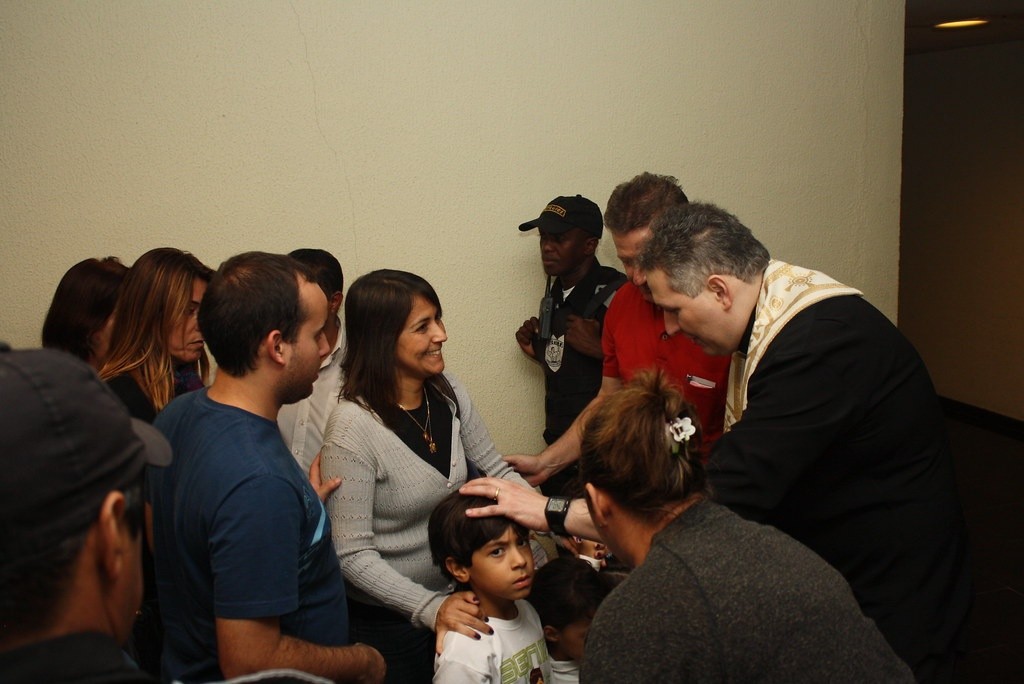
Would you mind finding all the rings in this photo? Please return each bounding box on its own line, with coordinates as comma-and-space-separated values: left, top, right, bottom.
494, 488, 500, 500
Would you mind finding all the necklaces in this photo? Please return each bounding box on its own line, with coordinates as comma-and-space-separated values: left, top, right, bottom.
396, 386, 437, 453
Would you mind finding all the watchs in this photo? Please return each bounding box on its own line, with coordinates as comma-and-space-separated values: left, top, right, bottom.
545, 497, 572, 538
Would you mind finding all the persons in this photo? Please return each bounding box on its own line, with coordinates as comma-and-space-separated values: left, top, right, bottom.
141, 251, 386, 684
507, 172, 729, 489
526, 557, 610, 684
578, 369, 919, 684
515, 195, 626, 556
320, 269, 607, 684
0, 349, 173, 684
97, 248, 217, 425
42, 256, 129, 370
277, 248, 347, 481
455, 203, 973, 684
428, 488, 552, 684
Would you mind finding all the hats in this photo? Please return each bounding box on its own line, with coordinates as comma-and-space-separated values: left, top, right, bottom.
519, 194, 603, 239
0, 340, 174, 562
